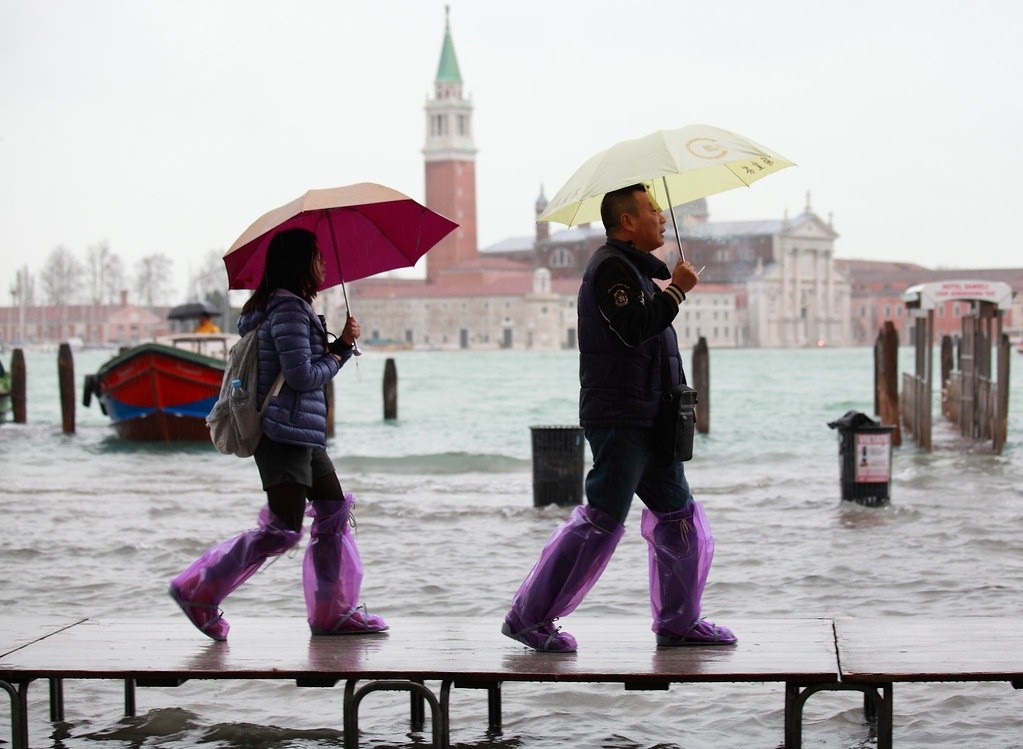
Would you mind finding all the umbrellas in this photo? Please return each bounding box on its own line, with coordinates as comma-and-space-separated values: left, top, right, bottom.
535, 123, 800, 263
222, 183, 460, 355
169, 301, 224, 321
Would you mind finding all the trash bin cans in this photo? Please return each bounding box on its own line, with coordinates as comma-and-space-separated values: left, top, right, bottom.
837, 424, 896, 508
530, 426, 584, 507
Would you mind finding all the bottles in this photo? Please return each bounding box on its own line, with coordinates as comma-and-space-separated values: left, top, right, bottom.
230, 380, 250, 409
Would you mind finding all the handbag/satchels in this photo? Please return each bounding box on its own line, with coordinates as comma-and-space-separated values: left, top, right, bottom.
661, 383, 698, 462
204, 299, 286, 458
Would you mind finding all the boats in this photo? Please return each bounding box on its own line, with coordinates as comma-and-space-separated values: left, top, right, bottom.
98, 341, 229, 442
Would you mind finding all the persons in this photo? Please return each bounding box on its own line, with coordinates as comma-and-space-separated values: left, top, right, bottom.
501, 183, 739, 652
193, 314, 219, 335
169, 228, 389, 640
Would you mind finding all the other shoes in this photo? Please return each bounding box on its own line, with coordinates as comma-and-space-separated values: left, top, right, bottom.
162, 575, 228, 644
311, 589, 388, 635
655, 618, 737, 650
500, 612, 572, 653
